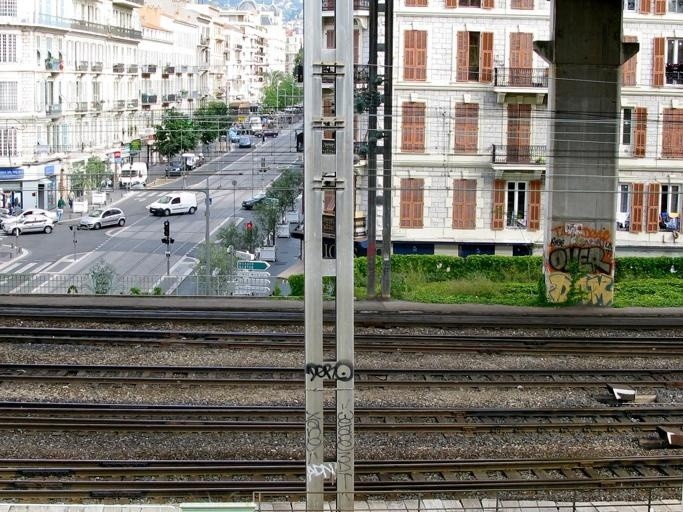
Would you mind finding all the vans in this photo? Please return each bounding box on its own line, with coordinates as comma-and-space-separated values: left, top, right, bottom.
149, 191, 197, 217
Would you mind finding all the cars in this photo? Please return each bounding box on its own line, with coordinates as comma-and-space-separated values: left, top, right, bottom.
0, 207, 58, 236
165, 153, 200, 177
242, 192, 267, 210
219, 116, 279, 149
80, 207, 126, 230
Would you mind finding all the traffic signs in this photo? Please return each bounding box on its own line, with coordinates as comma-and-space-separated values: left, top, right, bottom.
231, 250, 272, 295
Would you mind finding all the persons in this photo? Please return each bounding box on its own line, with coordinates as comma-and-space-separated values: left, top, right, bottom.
66, 189, 75, 209
7, 202, 13, 216
55, 195, 65, 215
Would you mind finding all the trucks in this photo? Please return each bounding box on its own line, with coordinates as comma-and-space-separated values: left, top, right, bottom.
119, 162, 148, 189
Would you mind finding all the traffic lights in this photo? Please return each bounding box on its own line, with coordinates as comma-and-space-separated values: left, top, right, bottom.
247, 223, 252, 239
161, 220, 175, 244
